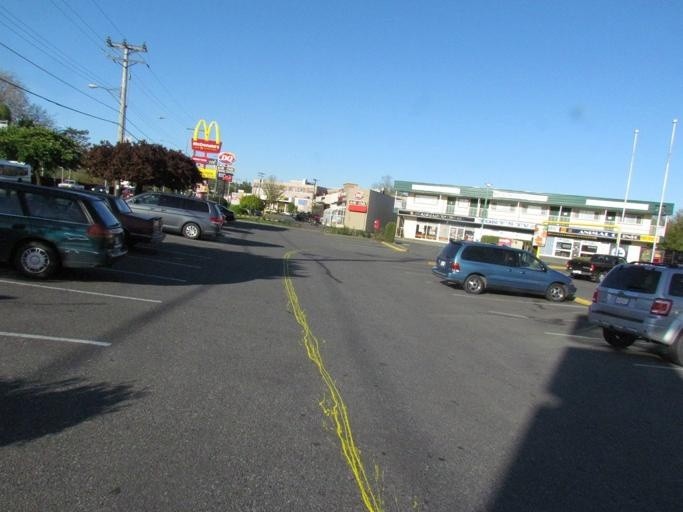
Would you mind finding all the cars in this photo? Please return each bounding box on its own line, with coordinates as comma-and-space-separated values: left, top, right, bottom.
56, 180, 135, 200
265, 208, 321, 224
216, 203, 236, 224
68, 187, 167, 249
242, 207, 262, 216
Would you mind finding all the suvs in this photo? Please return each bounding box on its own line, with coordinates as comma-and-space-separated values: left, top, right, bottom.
585, 260, 682, 364
563, 252, 630, 283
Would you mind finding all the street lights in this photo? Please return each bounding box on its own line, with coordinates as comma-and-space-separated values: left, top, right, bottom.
88, 82, 129, 144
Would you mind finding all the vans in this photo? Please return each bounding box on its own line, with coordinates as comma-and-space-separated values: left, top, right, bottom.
433, 237, 578, 302
0, 161, 32, 195
123, 191, 224, 242
0, 178, 128, 279
